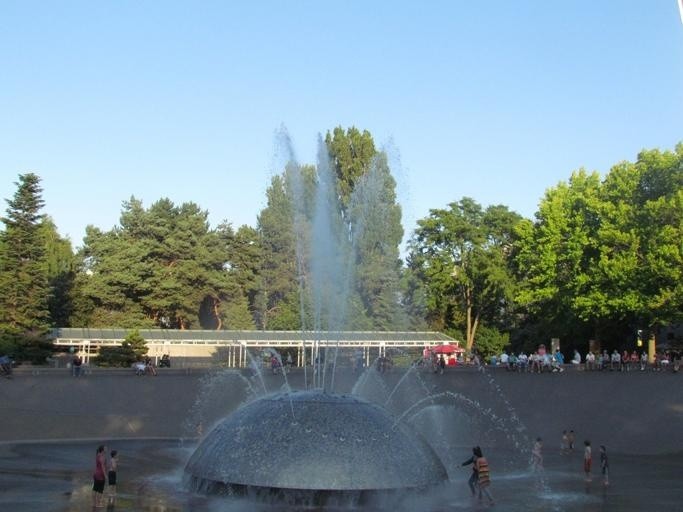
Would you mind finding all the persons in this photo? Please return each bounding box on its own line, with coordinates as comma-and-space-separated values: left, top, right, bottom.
92, 445, 107, 508
584, 440, 593, 482
250, 348, 393, 375
133, 354, 170, 376
107, 450, 119, 507
532, 437, 543, 469
421, 344, 683, 375
560, 430, 576, 456
72, 357, 82, 376
461, 446, 496, 505
600, 445, 609, 486
0, 352, 11, 375
